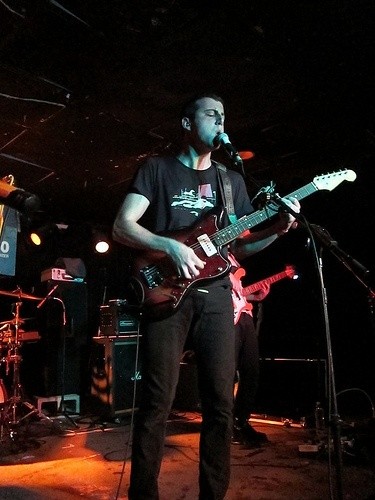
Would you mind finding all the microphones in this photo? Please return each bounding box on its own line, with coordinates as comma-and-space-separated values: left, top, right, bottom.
215, 133, 241, 162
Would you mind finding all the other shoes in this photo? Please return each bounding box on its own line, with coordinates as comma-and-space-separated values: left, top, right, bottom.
232, 418, 268, 443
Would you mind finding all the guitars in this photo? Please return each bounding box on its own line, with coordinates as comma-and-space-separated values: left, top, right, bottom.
126, 166, 355, 320
232, 262, 301, 325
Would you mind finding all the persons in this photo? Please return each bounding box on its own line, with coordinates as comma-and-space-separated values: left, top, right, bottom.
113, 93, 301, 500
227, 253, 270, 443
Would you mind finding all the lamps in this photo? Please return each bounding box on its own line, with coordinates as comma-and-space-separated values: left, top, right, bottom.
30, 224, 59, 246
93, 231, 111, 254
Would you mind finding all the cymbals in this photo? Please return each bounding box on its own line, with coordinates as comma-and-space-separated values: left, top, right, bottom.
0, 289, 42, 300
0, 317, 32, 324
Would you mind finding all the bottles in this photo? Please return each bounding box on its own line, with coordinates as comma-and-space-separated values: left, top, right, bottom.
315, 402, 329, 439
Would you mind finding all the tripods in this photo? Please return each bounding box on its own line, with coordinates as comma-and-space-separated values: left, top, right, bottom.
37, 283, 83, 428
0, 298, 54, 425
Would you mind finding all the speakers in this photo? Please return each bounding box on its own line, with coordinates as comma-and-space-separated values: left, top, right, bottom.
92, 341, 144, 416
